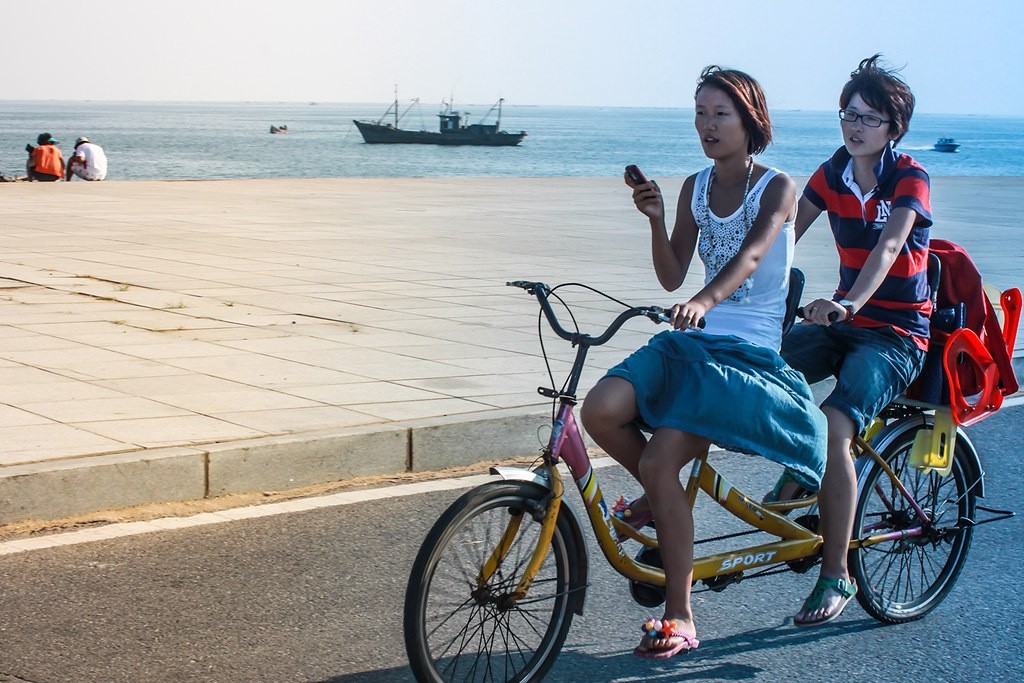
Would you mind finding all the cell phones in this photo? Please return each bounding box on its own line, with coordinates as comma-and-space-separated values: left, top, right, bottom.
626, 165, 659, 203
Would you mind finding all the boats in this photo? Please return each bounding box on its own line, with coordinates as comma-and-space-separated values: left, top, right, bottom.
270, 124, 288, 134
352, 84, 528, 147
933, 137, 961, 153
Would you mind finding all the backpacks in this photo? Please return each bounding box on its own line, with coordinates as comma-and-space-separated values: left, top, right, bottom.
927, 235, 1019, 398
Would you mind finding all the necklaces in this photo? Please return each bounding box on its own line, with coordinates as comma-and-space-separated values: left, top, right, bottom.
704, 156, 754, 305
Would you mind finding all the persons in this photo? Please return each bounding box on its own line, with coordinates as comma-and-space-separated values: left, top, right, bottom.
763, 54, 934, 628
26, 133, 66, 182
65, 136, 107, 182
580, 65, 828, 659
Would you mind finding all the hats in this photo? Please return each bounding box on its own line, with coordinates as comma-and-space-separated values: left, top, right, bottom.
47, 138, 58, 144
74, 137, 89, 149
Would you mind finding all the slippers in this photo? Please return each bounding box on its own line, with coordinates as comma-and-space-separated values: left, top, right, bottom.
612, 496, 652, 542
633, 619, 700, 659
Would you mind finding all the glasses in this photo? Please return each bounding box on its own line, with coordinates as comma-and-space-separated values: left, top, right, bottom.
839, 109, 891, 127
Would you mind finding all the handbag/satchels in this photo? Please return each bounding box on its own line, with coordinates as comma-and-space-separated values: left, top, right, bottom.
904, 254, 966, 405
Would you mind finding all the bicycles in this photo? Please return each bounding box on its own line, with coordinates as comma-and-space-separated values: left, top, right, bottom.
402, 253, 1016, 683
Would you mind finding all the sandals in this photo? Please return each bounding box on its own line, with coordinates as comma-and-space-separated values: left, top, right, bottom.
763, 467, 808, 516
794, 571, 859, 627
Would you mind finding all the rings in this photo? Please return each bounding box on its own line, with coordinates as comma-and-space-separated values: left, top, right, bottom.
813, 307, 818, 311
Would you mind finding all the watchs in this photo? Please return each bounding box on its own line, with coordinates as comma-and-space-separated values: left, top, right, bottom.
838, 299, 854, 322
